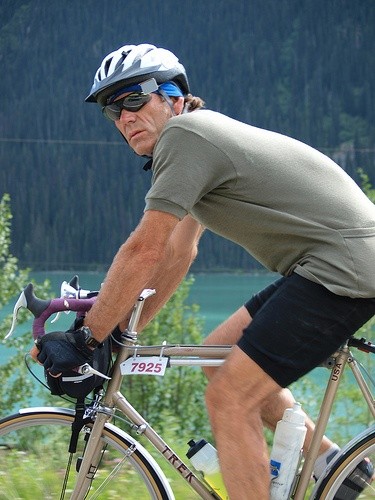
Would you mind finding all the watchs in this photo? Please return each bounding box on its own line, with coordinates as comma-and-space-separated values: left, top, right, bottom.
76, 325, 100, 348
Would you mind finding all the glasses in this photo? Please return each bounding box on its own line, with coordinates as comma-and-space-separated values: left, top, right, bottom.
100, 89, 150, 122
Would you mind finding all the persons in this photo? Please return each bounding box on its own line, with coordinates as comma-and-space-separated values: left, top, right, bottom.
29, 42, 375, 500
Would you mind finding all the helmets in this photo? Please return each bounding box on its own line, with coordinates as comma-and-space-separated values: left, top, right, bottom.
84, 44, 190, 108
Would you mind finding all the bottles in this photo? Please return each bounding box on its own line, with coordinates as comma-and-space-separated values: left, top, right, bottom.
185, 438, 230, 500
269, 401, 307, 500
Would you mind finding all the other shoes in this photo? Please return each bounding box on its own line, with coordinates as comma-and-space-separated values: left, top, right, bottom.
315, 457, 374, 499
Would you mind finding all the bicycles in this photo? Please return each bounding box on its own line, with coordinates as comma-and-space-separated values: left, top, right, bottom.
0, 274, 374, 500
